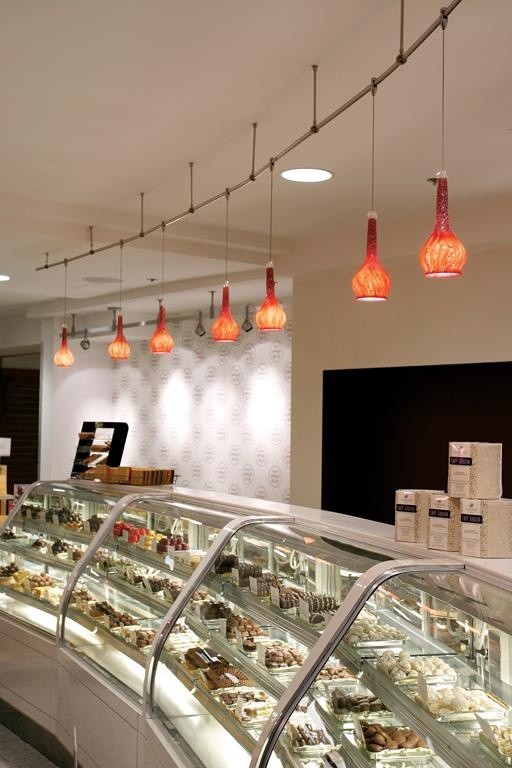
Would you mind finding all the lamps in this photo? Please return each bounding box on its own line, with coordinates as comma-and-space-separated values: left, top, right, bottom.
255, 157, 286, 333
149, 223, 173, 354
212, 189, 240, 343
351, 85, 393, 302
107, 241, 130, 360
54, 257, 75, 367
419, 13, 467, 279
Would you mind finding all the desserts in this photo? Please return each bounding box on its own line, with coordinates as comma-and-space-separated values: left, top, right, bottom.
1, 503, 512, 768
